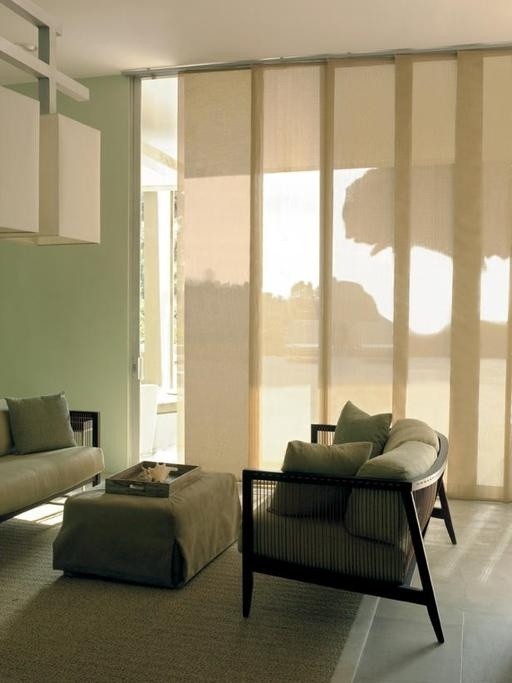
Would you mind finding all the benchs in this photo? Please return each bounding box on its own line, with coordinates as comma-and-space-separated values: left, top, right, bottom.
52, 469, 243, 590
1, 445, 105, 525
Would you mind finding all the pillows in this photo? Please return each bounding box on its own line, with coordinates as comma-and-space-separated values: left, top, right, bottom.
265, 400, 442, 545
1, 389, 78, 457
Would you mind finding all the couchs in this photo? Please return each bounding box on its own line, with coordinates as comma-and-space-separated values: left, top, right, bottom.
236, 398, 457, 643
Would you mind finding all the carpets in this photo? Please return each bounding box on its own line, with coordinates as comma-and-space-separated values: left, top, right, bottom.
0, 515, 382, 683
